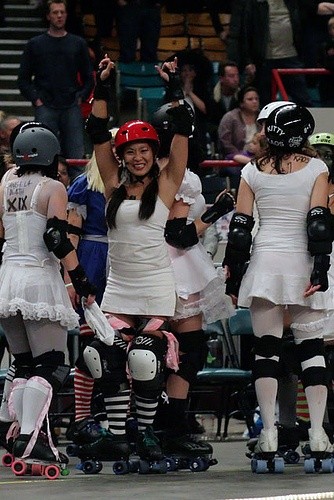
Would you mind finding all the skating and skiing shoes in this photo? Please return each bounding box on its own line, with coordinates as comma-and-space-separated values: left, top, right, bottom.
246, 418, 301, 463
131, 426, 166, 475
304, 427, 334, 473
10, 432, 69, 480
104, 430, 140, 475
0, 421, 22, 466
250, 427, 285, 473
65, 418, 108, 474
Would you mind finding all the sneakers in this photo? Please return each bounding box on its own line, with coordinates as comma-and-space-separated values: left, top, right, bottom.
163, 433, 216, 472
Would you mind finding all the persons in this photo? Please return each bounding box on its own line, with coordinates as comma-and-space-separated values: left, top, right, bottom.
0, 125, 96, 477
165, 47, 278, 201
222, 100, 334, 475
234, 127, 264, 164
150, 102, 234, 472
82, 14, 98, 38
65, 122, 123, 299
226, 0, 334, 106
113, 0, 165, 63
53, 160, 72, 189
19, 0, 94, 178
75, 54, 194, 473
309, 132, 334, 168
0, 112, 17, 159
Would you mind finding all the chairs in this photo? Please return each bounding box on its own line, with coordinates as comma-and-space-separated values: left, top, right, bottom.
99, 12, 233, 122
193, 308, 253, 441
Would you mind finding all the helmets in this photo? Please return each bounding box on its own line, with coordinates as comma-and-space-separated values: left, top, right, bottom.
10, 122, 53, 149
256, 101, 296, 122
12, 128, 62, 166
114, 120, 159, 150
264, 104, 315, 150
